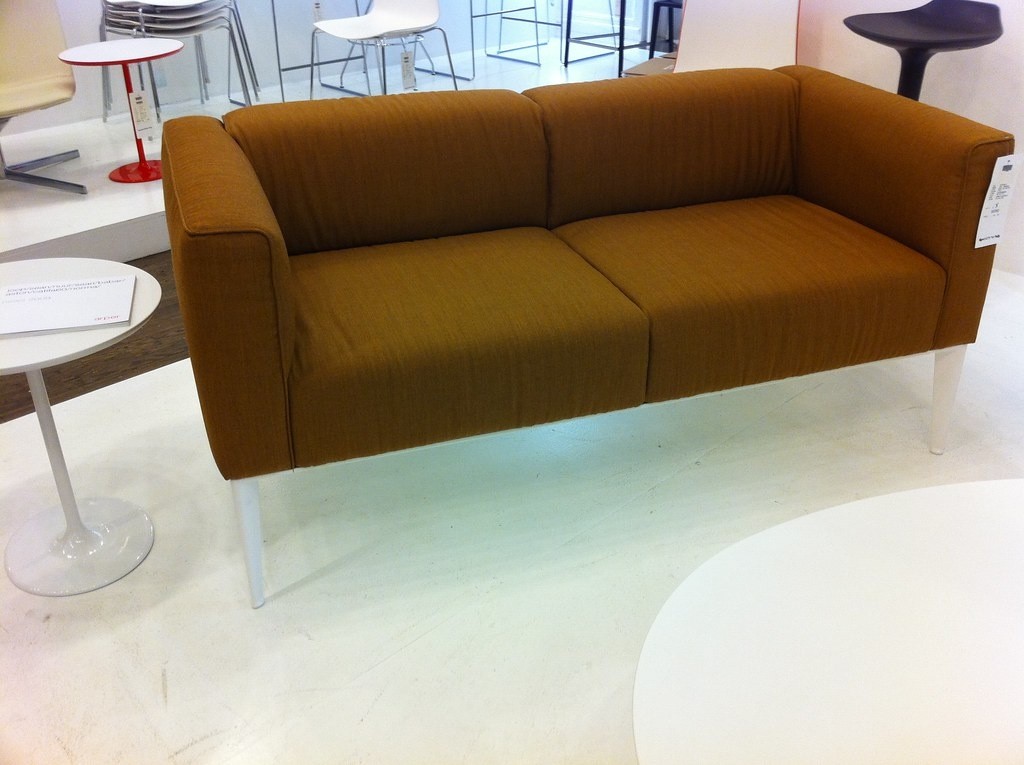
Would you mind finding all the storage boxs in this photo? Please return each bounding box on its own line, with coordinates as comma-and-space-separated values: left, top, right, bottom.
621, 57, 676, 78
659, 51, 678, 59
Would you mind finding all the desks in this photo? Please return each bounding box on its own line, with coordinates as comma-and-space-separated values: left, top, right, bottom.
58, 37, 184, 183
0, 258, 162, 597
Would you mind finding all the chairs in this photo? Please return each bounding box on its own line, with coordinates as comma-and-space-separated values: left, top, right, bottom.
0, 0, 88, 195
98, 0, 684, 124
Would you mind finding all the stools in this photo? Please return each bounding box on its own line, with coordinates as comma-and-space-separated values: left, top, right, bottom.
843, 0, 1003, 101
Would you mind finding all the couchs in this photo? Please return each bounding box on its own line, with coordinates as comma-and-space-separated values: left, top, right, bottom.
160, 65, 1015, 478
